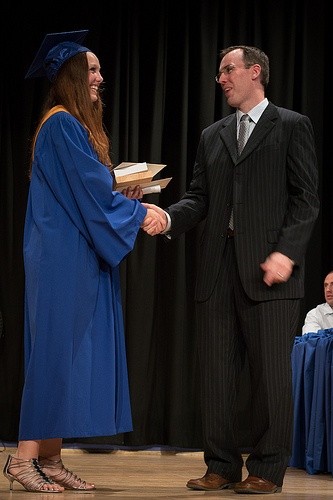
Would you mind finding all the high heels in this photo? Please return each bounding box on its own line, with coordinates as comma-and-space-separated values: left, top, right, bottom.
37, 452, 95, 490
3, 454, 62, 493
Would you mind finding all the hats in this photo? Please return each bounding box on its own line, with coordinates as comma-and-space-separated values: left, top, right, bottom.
23, 29, 90, 82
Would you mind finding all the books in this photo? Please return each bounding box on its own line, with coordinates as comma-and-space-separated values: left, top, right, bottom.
112, 162, 153, 192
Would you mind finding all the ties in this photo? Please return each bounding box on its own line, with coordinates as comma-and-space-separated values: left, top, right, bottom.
229, 115, 250, 231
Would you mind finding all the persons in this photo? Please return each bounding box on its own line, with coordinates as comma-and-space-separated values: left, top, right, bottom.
137, 45, 320, 494
3, 29, 169, 493
302, 271, 333, 335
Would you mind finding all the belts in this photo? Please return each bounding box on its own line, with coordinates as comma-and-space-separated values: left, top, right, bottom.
227, 231, 234, 239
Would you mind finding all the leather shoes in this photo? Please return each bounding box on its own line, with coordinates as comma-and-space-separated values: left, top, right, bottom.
234, 476, 283, 493
186, 470, 243, 491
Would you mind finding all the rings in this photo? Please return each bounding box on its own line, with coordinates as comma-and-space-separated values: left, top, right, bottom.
276, 272, 284, 277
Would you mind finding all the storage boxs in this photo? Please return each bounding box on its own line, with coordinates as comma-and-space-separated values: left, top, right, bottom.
114, 171, 153, 191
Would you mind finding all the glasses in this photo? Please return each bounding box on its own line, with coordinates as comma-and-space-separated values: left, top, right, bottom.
215, 65, 253, 83
